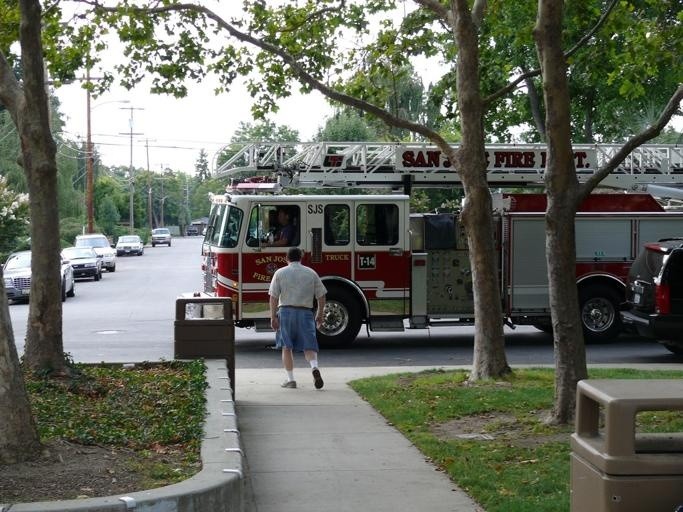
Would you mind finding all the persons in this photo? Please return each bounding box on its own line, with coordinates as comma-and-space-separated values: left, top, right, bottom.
258, 209, 299, 247
267, 247, 328, 389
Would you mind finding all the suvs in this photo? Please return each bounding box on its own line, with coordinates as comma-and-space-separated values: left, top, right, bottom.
72, 233, 115, 272
149, 228, 173, 248
618, 235, 682, 355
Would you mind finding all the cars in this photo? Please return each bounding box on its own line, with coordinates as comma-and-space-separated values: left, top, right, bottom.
60, 246, 103, 281
114, 235, 143, 256
0, 249, 75, 305
185, 226, 198, 236
201, 228, 206, 236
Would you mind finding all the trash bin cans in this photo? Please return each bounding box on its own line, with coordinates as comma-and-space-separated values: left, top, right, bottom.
175, 297, 234, 407
570, 379, 681, 512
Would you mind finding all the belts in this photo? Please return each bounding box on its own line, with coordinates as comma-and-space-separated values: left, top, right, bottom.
279, 306, 312, 309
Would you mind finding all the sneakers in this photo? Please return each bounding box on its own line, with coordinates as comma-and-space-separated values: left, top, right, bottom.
312, 367, 323, 389
281, 380, 296, 388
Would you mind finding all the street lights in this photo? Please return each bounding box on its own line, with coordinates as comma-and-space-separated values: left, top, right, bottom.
86, 96, 131, 233
200, 141, 682, 349
